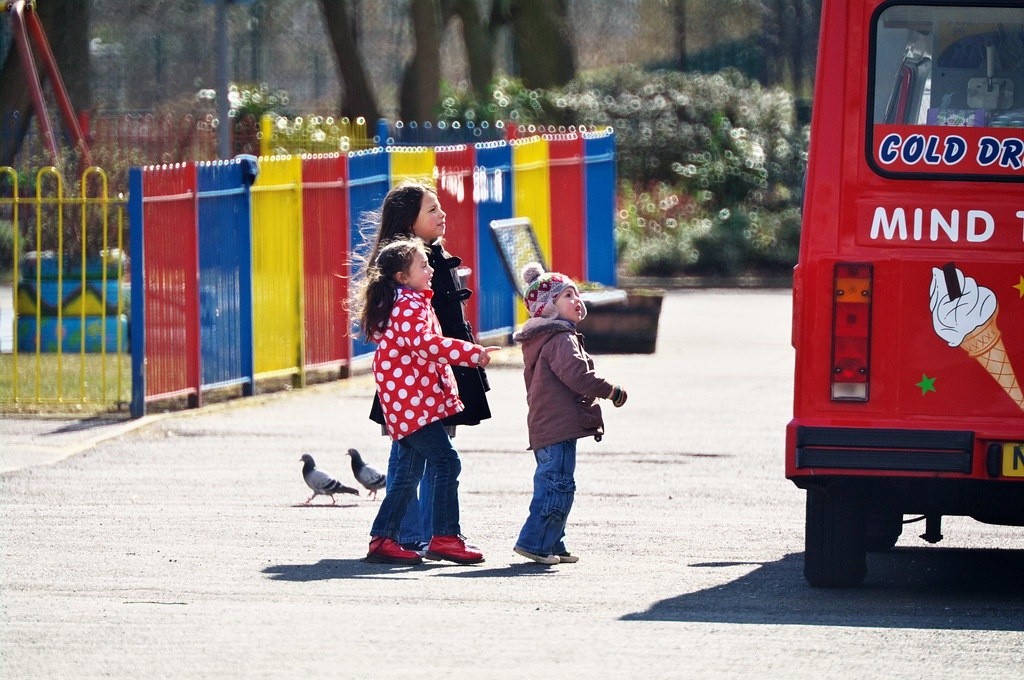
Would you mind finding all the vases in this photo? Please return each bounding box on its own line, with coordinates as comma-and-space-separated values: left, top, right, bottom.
579, 289, 663, 355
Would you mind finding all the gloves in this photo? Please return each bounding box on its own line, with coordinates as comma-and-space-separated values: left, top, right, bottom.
607, 385, 628, 409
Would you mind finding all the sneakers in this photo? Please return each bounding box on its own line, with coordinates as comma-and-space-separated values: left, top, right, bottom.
399, 541, 429, 557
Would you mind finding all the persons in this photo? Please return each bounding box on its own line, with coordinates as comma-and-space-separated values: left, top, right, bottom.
343, 239, 501, 566
367, 180, 492, 557
511, 260, 628, 565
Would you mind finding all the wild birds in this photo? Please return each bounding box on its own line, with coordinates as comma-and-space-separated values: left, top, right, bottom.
298, 454, 359, 506
345, 448, 387, 502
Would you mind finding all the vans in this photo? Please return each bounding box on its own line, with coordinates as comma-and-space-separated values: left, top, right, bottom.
784, 0, 1024, 590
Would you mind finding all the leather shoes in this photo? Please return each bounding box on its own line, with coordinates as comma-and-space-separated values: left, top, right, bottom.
359, 534, 422, 565
424, 535, 485, 565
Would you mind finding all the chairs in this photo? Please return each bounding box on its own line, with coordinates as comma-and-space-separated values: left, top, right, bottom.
490, 216, 547, 300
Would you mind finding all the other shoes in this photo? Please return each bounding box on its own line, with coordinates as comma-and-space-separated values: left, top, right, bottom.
555, 551, 579, 563
513, 545, 561, 564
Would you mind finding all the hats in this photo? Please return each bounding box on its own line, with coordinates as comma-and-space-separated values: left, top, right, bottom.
523, 262, 587, 322
